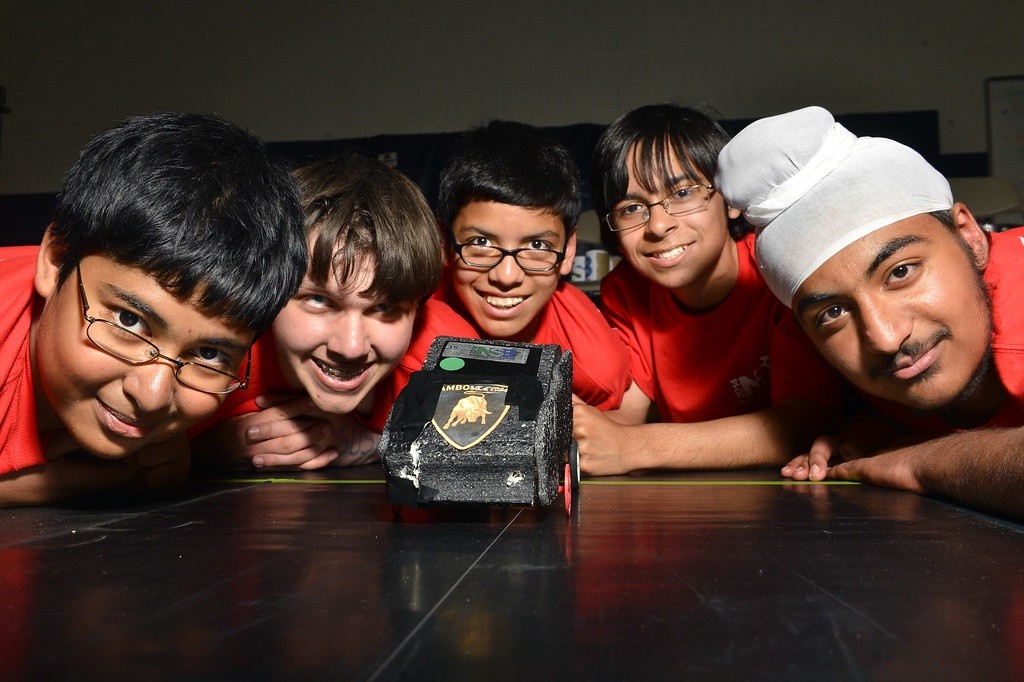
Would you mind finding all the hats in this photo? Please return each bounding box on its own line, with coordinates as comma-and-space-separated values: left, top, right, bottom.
714, 106, 953, 308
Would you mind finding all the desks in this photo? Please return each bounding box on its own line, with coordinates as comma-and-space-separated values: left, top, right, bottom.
0, 461, 1024, 682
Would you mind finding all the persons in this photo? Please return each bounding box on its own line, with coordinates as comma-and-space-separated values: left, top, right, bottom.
0, 113, 308, 505
372, 121, 633, 434
571, 102, 831, 476
219, 151, 482, 470
715, 106, 1024, 515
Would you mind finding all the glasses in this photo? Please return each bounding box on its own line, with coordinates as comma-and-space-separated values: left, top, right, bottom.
76, 262, 251, 395
605, 184, 713, 231
448, 228, 569, 276
